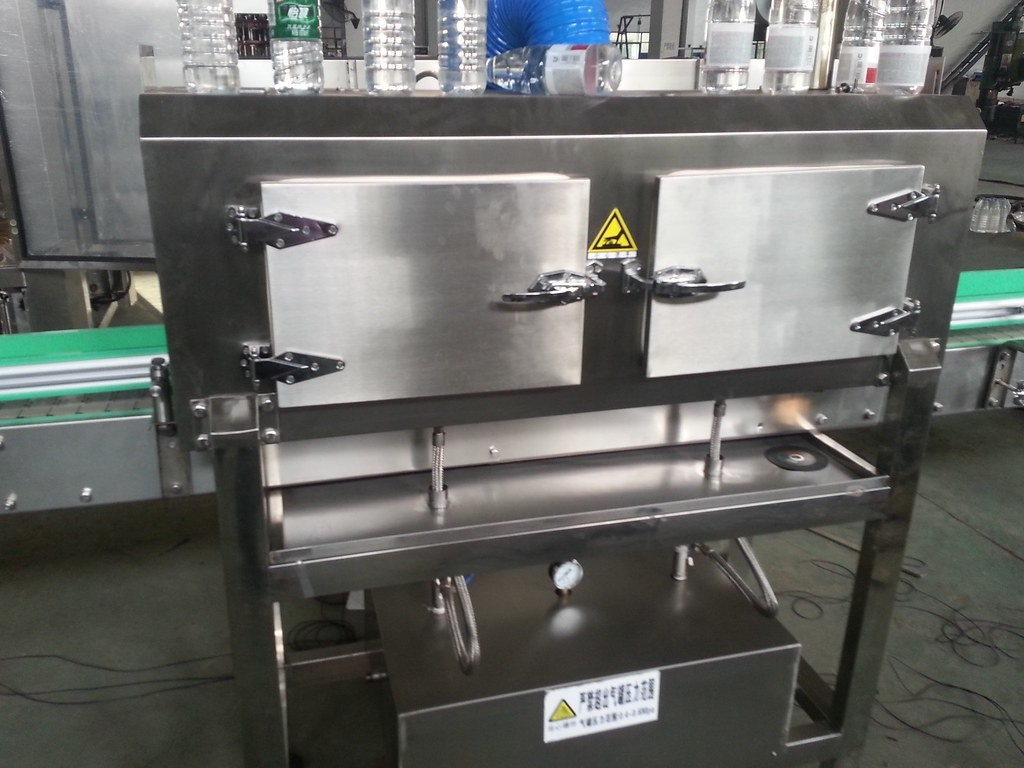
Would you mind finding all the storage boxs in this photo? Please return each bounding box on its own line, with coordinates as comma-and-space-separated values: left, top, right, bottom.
365, 543, 801, 767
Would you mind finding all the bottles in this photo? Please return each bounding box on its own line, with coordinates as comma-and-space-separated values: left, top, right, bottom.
762, 0, 824, 96
361, 0, 416, 97
175, 0, 241, 94
266, 0, 325, 97
876, 0, 936, 95
437, 0, 487, 95
837, 0, 888, 92
486, 43, 622, 97
700, 0, 757, 97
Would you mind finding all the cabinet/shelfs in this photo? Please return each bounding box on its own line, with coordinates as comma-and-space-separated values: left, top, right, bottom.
133, 89, 987, 767
